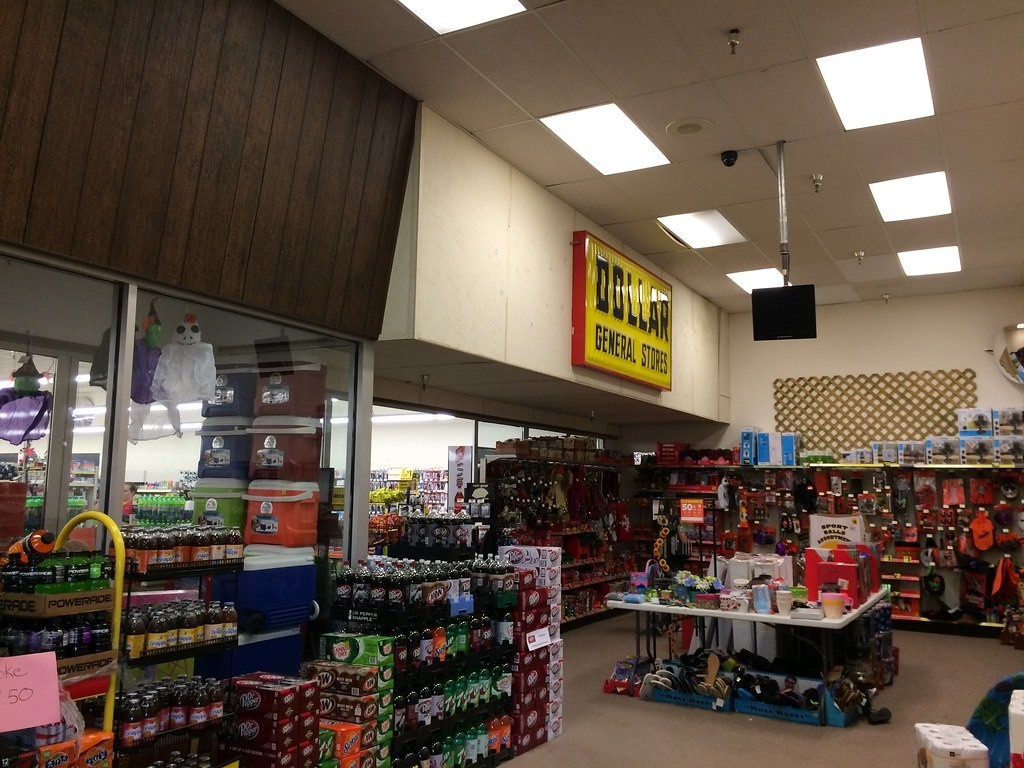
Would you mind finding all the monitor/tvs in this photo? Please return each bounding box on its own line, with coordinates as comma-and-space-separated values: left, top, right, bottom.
751, 284, 816, 341
256, 341, 294, 378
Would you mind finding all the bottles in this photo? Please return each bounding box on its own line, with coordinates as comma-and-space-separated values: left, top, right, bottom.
69, 458, 95, 471
370, 504, 474, 551
150, 750, 213, 768
7, 529, 56, 564
23, 495, 45, 528
332, 552, 517, 768
0, 550, 109, 595
0, 552, 7, 568
1, 612, 110, 660
84, 674, 224, 748
120, 597, 238, 662
109, 493, 244, 574
66, 495, 86, 529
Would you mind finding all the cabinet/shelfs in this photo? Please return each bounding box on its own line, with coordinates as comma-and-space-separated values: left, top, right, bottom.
101, 557, 245, 757
547, 529, 631, 625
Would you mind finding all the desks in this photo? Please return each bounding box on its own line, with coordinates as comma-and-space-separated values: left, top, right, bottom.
605, 587, 888, 727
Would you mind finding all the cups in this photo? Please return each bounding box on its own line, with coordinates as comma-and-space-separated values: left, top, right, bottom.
821, 592, 844, 618
776, 590, 793, 616
719, 579, 753, 612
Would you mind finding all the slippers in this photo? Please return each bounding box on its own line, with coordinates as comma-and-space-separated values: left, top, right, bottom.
639, 647, 892, 727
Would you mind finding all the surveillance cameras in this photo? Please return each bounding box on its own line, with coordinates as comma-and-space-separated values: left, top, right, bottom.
721, 150, 737, 167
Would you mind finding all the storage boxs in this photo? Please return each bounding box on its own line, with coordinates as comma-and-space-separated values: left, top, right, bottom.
652, 663, 872, 727
804, 513, 883, 610
496, 546, 561, 756
632, 469, 723, 577
0, 589, 115, 618
56, 648, 116, 682
192, 359, 328, 680
656, 405, 1024, 465
496, 437, 597, 464
